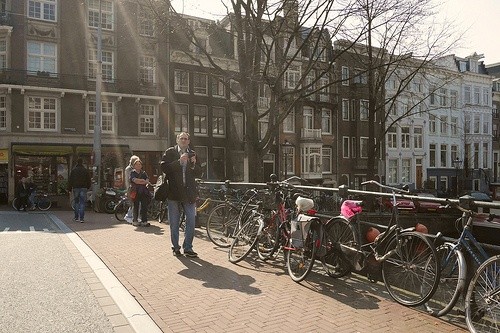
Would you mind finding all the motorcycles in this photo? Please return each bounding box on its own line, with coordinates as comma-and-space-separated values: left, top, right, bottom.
103, 187, 128, 213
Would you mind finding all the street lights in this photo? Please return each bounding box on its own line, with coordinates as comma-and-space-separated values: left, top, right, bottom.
455, 157, 459, 197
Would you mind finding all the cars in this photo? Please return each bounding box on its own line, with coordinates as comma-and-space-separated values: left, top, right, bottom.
459, 192, 494, 202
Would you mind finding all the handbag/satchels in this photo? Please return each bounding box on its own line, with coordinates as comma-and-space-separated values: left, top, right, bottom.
130, 191, 137, 201
309, 220, 327, 257
154, 176, 169, 200
291, 213, 312, 248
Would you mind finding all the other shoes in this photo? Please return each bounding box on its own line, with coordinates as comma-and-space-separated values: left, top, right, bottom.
133, 221, 141, 226
174, 249, 181, 256
184, 250, 198, 256
140, 221, 150, 227
73, 218, 79, 221
80, 219, 84, 223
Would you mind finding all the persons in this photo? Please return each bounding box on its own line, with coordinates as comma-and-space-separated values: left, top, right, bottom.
17, 177, 36, 211
124, 156, 150, 226
68, 159, 91, 223
157, 132, 202, 256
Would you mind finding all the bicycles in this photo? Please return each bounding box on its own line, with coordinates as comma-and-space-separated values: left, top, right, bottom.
11, 192, 53, 211
71, 190, 95, 212
179, 184, 265, 263
420, 197, 500, 333
321, 179, 439, 307
115, 189, 185, 227
257, 176, 322, 283
